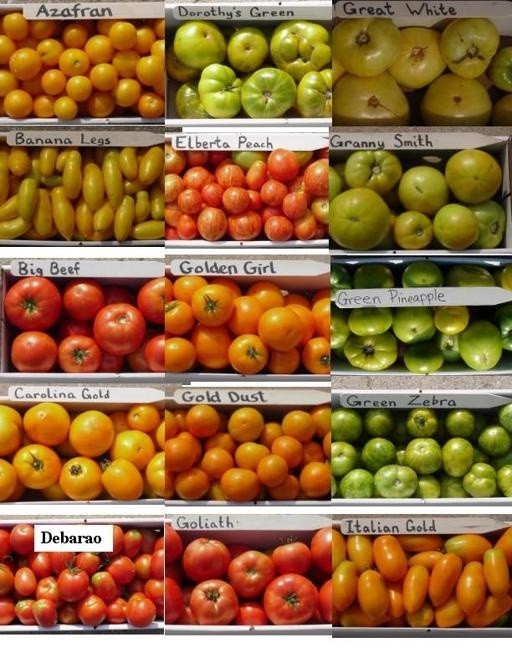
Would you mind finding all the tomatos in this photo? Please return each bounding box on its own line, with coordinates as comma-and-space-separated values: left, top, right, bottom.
0, 0, 512, 628
